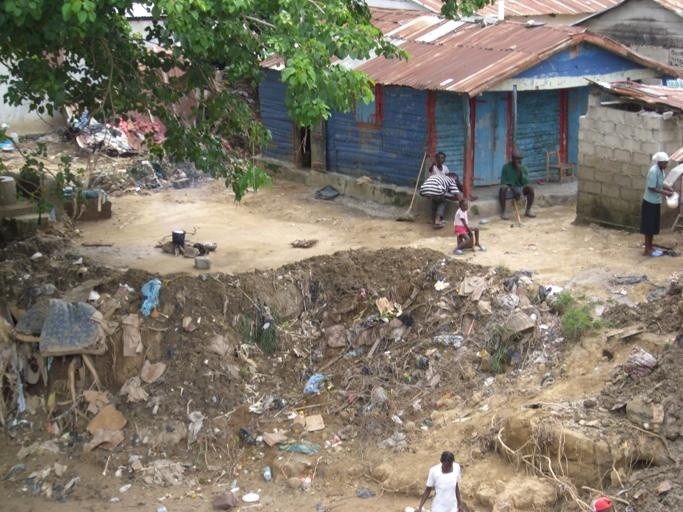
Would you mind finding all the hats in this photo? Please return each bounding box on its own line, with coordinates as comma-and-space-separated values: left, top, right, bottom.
652, 152, 669, 162
512, 152, 523, 159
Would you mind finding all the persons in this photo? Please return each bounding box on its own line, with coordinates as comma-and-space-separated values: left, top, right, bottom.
591, 498, 614, 512
414, 451, 464, 512
454, 200, 487, 255
498, 150, 537, 219
429, 153, 478, 200
419, 173, 467, 229
641, 151, 674, 257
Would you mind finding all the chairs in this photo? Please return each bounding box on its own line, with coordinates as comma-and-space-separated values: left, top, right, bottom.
545, 149, 578, 184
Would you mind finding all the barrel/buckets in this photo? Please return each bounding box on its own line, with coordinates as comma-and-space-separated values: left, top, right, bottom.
172, 230, 186, 244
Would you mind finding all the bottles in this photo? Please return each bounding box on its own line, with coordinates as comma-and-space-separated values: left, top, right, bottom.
263, 466, 272, 481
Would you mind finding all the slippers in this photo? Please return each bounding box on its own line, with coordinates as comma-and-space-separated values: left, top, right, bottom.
643, 249, 664, 257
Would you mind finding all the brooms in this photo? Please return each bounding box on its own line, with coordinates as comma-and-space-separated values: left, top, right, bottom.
396, 151, 427, 221
